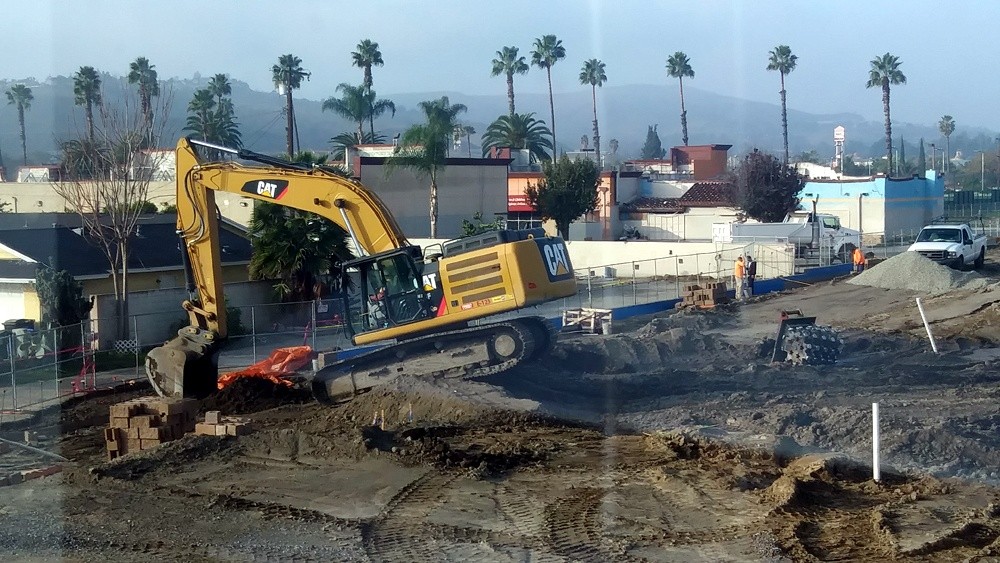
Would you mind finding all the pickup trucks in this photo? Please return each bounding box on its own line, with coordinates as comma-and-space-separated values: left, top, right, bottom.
907, 225, 986, 271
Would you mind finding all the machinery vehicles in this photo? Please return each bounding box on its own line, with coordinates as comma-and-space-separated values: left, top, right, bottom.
145, 136, 578, 408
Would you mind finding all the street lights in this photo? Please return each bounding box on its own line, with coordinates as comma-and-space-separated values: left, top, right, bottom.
976, 150, 984, 197
937, 148, 945, 173
858, 193, 869, 249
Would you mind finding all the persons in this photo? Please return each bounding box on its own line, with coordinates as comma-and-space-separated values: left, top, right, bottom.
850, 248, 865, 275
735, 256, 756, 302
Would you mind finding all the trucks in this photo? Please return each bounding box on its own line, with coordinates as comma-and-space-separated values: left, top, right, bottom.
712, 211, 862, 263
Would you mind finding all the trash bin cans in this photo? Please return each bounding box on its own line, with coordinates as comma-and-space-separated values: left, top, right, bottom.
11, 328, 34, 359
0, 329, 9, 359
3, 318, 35, 334
